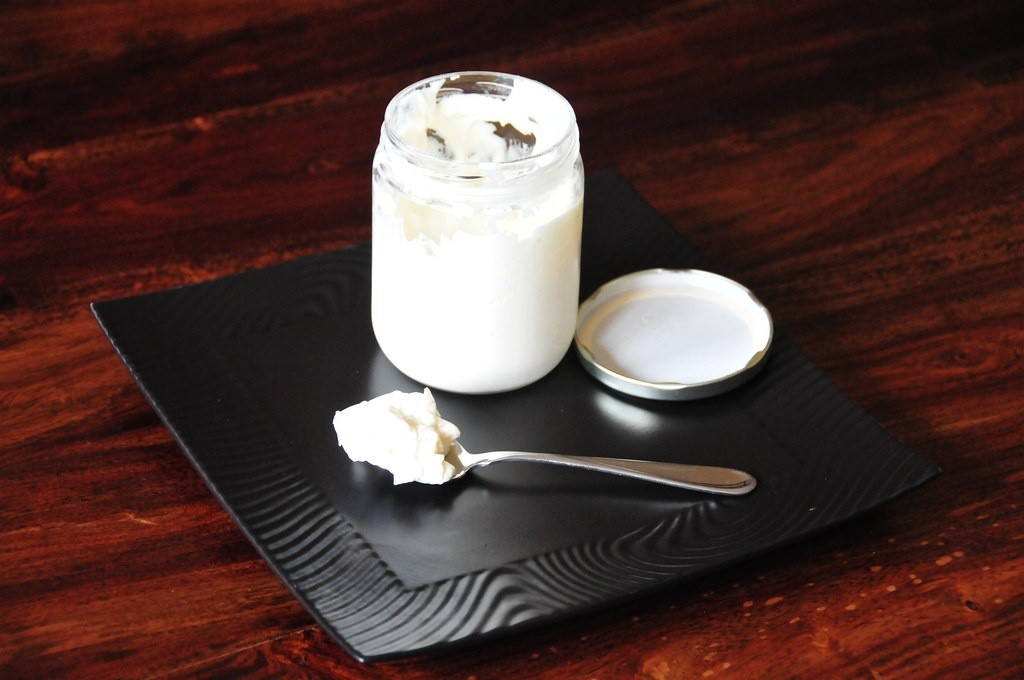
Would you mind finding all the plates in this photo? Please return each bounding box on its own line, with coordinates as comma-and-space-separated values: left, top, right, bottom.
90, 167, 945, 666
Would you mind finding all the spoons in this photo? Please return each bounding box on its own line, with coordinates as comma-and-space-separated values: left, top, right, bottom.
443, 441, 757, 496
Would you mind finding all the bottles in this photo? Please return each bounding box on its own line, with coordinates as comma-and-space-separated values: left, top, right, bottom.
370, 71, 586, 396
